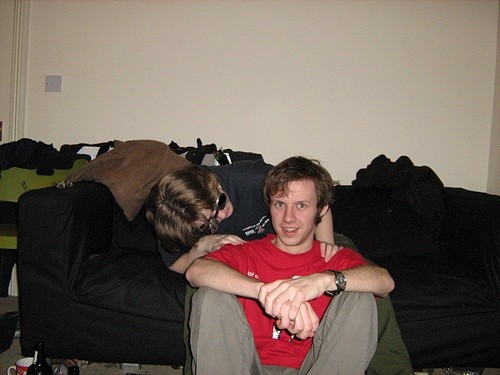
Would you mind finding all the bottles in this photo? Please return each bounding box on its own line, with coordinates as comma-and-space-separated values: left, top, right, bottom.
26, 342, 52, 375
68, 366, 79, 375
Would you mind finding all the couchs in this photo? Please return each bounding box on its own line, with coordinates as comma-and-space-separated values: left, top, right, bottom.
17, 180, 500, 370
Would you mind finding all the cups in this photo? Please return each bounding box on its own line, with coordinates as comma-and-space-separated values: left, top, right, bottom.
7, 357, 33, 375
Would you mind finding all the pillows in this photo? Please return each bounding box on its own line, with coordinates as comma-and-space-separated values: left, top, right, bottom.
67, 140, 190, 222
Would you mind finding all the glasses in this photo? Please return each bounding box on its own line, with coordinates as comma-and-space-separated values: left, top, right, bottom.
206, 193, 227, 235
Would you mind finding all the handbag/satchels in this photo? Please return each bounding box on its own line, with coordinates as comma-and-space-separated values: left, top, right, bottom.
64, 140, 189, 222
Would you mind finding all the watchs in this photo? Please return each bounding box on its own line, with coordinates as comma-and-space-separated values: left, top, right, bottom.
325, 270, 347, 296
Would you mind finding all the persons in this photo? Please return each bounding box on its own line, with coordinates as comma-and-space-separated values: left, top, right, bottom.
151, 159, 343, 276
185, 155, 395, 375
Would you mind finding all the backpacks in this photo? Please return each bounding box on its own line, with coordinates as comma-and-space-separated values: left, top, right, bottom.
352, 155, 444, 260
